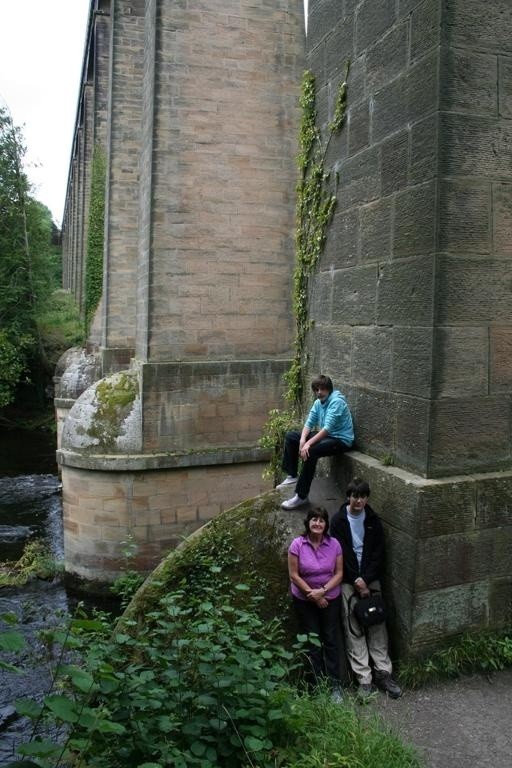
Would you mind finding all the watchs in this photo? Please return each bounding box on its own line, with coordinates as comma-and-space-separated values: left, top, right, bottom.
322, 584, 328, 596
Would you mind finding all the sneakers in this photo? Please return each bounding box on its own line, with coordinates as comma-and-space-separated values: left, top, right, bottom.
327, 686, 343, 707
356, 683, 372, 700
276, 474, 298, 489
376, 676, 403, 697
281, 492, 309, 509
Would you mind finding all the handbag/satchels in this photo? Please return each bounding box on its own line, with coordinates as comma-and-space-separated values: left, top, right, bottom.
348, 589, 386, 637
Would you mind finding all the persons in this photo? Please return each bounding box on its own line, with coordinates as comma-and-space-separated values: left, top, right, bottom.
327, 477, 405, 704
273, 371, 358, 511
284, 505, 347, 711
